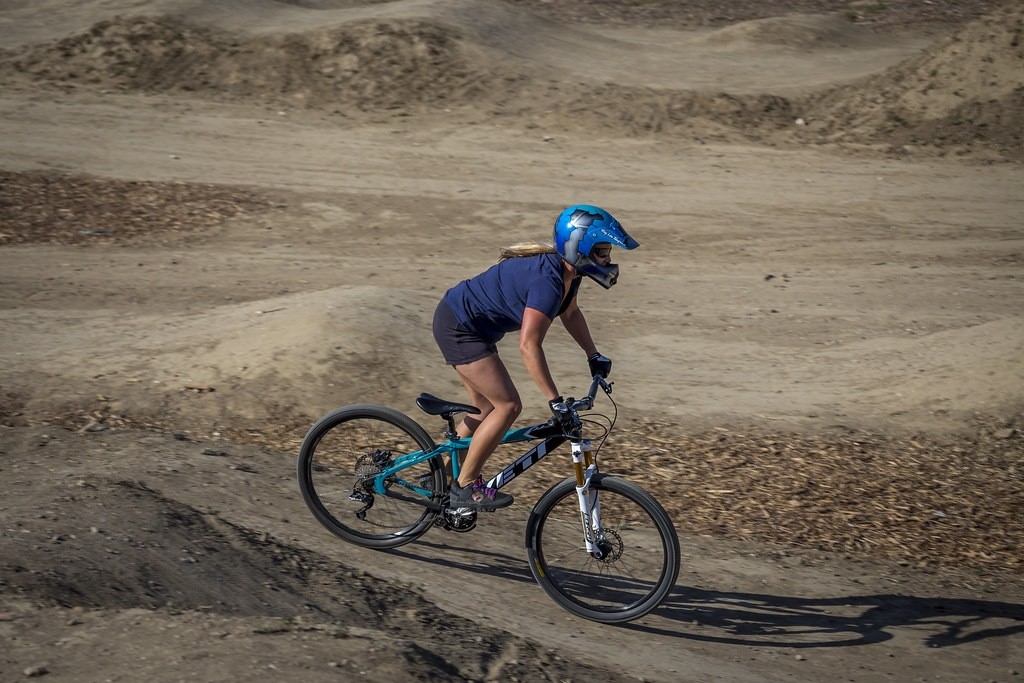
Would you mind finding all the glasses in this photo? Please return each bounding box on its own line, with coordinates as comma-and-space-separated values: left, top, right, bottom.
593, 247, 613, 257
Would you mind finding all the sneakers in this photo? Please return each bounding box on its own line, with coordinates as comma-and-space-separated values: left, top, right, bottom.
418, 473, 436, 502
450, 479, 514, 510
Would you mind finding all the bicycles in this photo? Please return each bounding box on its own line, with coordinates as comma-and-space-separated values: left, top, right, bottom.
297, 372, 681, 625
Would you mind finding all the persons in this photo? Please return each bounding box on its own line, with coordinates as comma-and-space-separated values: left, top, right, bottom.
432, 204, 640, 508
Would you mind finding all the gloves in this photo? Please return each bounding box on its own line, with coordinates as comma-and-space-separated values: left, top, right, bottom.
549, 395, 580, 434
587, 354, 612, 380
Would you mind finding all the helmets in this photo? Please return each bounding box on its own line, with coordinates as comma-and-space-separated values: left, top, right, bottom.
552, 204, 641, 290
